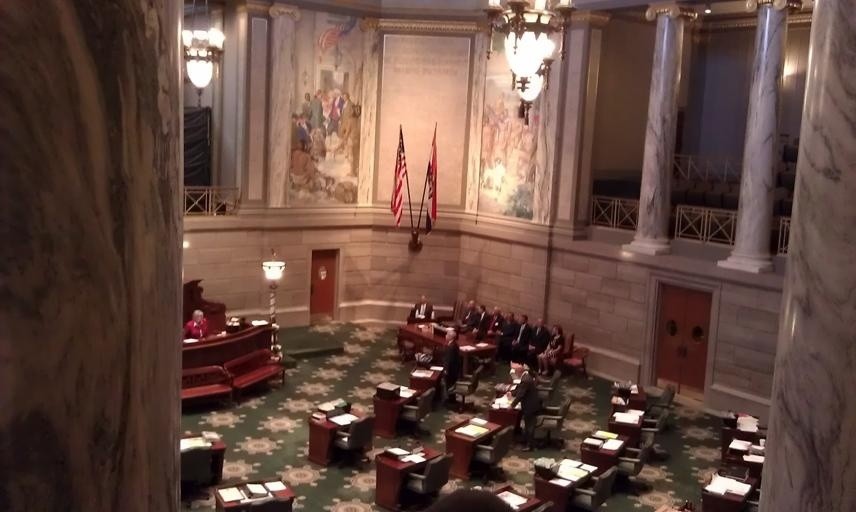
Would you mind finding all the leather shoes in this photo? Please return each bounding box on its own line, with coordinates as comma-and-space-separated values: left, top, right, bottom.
521, 447, 531, 452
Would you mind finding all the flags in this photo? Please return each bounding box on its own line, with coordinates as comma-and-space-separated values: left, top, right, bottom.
390, 125, 408, 226
425, 121, 438, 237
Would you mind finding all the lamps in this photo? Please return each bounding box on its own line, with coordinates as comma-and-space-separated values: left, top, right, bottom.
485, 0, 577, 126
183, 1, 225, 94
263, 262, 286, 362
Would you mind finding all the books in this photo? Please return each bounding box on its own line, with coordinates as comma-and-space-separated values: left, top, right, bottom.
384, 448, 410, 460
583, 438, 603, 450
202, 431, 220, 442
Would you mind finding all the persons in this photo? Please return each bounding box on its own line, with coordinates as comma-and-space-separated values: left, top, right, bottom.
457, 299, 479, 332
291, 113, 301, 147
327, 89, 345, 136
346, 105, 360, 177
510, 314, 531, 357
301, 93, 311, 115
486, 306, 505, 339
411, 294, 433, 324
538, 324, 565, 375
309, 89, 326, 130
500, 311, 518, 342
184, 309, 209, 340
506, 375, 544, 452
340, 93, 354, 136
443, 329, 460, 409
471, 304, 491, 339
291, 139, 317, 190
519, 317, 551, 372
298, 113, 313, 153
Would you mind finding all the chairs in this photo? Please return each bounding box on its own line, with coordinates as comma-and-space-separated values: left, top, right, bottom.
647, 384, 676, 420
180, 444, 215, 507
241, 496, 290, 512
180, 365, 232, 412
224, 348, 285, 405
562, 347, 589, 377
334, 414, 373, 470
615, 433, 654, 496
403, 454, 449, 510
532, 501, 554, 512
472, 426, 514, 484
639, 408, 669, 453
449, 365, 484, 413
560, 333, 573, 358
572, 466, 617, 512
536, 399, 571, 449
399, 387, 436, 440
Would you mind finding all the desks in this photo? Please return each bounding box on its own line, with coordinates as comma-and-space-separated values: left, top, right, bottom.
721, 420, 753, 462
444, 417, 500, 481
182, 323, 271, 373
400, 321, 499, 374
740, 445, 765, 485
491, 484, 541, 512
308, 406, 363, 466
581, 433, 630, 479
613, 389, 648, 417
701, 471, 757, 512
534, 459, 600, 512
408, 367, 444, 403
372, 385, 421, 440
609, 410, 646, 447
180, 433, 225, 482
215, 478, 294, 512
488, 397, 524, 440
375, 447, 441, 511
